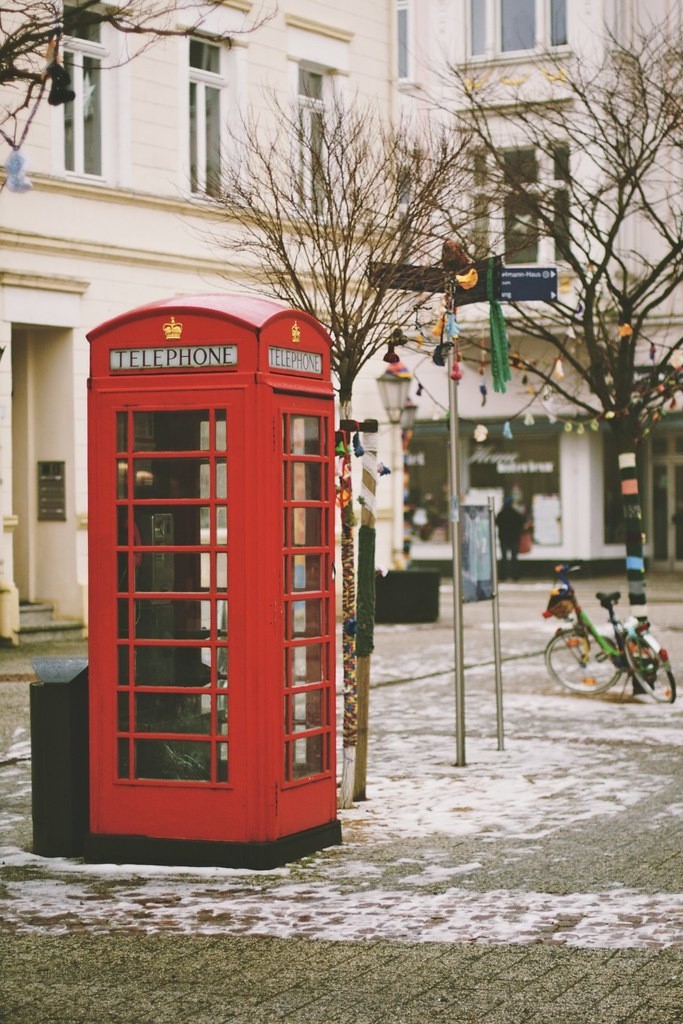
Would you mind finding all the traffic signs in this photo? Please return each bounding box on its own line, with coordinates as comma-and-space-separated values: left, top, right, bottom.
500, 266, 557, 301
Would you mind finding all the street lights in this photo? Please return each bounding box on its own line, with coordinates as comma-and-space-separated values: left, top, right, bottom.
375, 371, 410, 569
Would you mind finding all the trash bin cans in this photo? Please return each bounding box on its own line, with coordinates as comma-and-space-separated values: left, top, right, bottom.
29, 653, 92, 860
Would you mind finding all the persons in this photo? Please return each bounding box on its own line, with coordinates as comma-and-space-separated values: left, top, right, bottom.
493, 497, 527, 580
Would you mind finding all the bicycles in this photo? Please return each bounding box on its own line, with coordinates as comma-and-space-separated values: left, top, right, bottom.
542, 563, 677, 704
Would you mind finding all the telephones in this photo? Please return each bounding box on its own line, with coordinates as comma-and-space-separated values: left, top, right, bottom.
118, 511, 180, 608
